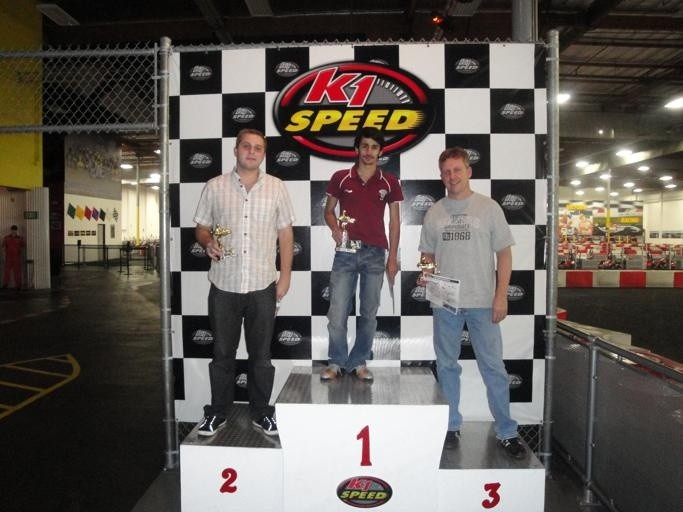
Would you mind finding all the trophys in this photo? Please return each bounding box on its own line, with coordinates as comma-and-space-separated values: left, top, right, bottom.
210, 224, 235, 260
419, 256, 440, 274
335, 210, 356, 253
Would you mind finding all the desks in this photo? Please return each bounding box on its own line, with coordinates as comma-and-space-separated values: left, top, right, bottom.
76, 240, 147, 273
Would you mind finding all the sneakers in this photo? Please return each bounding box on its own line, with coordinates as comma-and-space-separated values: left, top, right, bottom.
447, 428, 461, 450
253, 416, 280, 436
321, 364, 339, 380
501, 437, 526, 458
352, 364, 373, 381
198, 415, 227, 436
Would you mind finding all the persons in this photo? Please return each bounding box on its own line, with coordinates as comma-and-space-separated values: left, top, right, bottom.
192, 129, 296, 437
320, 126, 405, 381
0, 225, 25, 292
417, 148, 529, 459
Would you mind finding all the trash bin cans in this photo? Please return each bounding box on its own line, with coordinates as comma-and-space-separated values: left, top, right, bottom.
24, 259, 34, 290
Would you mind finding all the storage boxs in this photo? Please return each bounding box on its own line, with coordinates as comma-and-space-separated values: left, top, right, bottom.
556, 307, 568, 320
